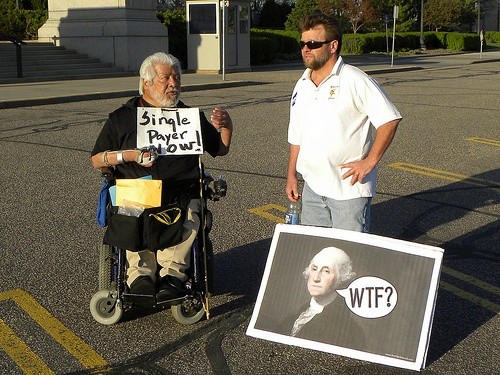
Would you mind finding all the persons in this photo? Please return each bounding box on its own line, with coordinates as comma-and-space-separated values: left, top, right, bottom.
89, 51, 234, 301
284, 13, 404, 233
278, 246, 369, 351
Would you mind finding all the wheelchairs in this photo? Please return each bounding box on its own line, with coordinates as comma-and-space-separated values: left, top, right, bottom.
90, 156, 212, 325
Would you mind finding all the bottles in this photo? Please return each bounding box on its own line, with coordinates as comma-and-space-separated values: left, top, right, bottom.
286, 197, 299, 224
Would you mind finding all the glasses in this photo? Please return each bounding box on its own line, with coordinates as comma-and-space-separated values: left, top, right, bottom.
299, 40, 334, 49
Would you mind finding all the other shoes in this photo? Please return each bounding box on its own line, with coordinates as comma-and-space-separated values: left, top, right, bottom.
130, 275, 156, 294
155, 275, 187, 302
